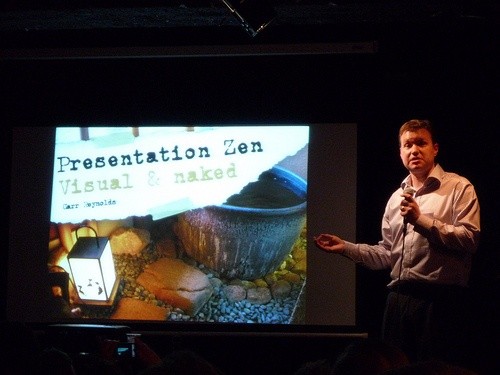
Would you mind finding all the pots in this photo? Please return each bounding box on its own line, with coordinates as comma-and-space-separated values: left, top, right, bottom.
177, 166, 308, 282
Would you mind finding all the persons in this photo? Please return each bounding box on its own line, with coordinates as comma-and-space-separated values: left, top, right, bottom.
0, 320, 223, 375
313, 120, 480, 375
297, 336, 478, 375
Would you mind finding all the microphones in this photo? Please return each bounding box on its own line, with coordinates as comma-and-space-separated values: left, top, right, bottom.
402, 188, 414, 236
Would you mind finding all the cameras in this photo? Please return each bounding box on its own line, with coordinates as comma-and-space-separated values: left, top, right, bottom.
114, 342, 136, 358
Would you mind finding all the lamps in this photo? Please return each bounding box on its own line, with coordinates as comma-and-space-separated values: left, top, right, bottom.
223, 0, 276, 37
